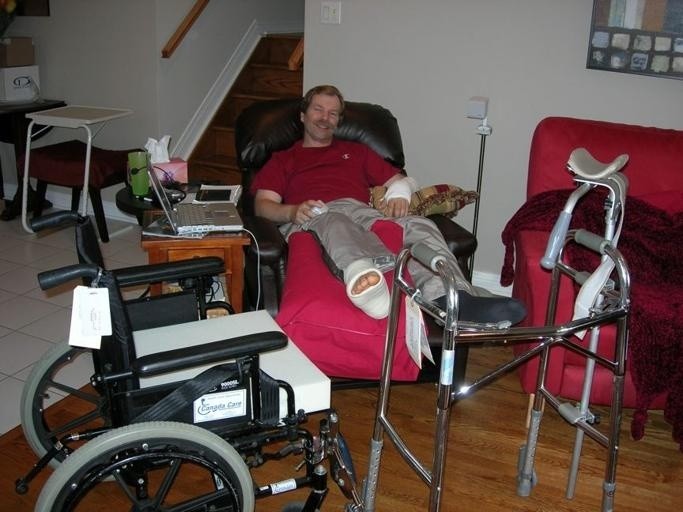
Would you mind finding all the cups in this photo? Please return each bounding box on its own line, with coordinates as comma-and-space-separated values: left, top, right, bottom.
127, 152, 151, 196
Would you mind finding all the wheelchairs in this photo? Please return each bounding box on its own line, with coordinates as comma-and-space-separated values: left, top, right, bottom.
14, 209, 373, 511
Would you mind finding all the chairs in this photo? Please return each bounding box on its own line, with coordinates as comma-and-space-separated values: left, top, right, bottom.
234, 96, 476, 390
511, 117, 683, 429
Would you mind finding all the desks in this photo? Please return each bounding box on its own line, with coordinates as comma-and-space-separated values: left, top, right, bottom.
0, 99, 69, 221
116, 180, 251, 313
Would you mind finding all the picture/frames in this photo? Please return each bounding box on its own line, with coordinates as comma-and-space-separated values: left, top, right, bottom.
585, 0, 683, 80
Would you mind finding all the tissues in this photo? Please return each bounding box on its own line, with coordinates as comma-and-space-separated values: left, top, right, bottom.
127, 135, 187, 190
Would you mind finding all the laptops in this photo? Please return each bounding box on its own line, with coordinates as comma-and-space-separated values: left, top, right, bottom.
145, 163, 244, 236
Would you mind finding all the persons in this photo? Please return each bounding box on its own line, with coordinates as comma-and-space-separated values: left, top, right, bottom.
245, 83, 529, 333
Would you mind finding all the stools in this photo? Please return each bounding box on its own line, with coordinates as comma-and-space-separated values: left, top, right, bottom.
20, 104, 146, 242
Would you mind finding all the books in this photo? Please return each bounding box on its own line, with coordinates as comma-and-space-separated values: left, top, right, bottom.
141, 214, 207, 240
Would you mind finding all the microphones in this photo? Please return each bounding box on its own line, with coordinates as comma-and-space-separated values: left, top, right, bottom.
130, 165, 173, 185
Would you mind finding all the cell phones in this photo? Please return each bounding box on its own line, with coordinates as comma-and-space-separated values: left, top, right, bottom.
195, 189, 232, 201
